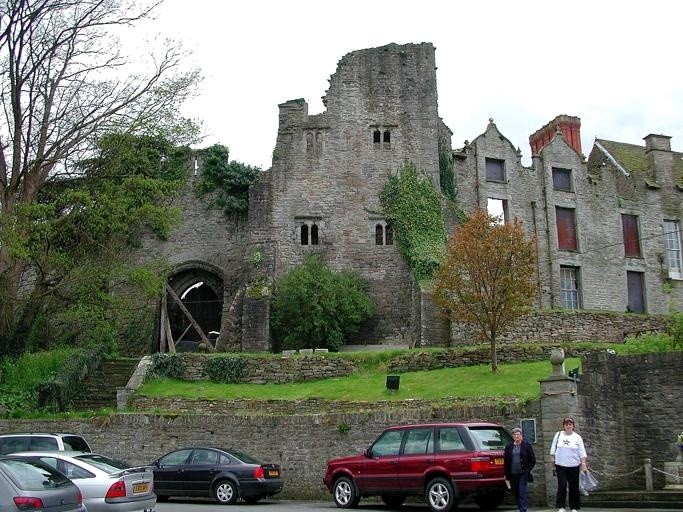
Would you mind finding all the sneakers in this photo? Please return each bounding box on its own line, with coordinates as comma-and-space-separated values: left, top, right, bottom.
558, 508, 566, 512
572, 509, 577, 512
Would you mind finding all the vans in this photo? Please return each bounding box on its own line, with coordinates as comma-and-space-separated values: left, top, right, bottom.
0, 433, 92, 456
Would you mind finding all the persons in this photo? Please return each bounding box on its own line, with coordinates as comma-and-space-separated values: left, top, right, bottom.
550, 416, 587, 512
500, 428, 535, 511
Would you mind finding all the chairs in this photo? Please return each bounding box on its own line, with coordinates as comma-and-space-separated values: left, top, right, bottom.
2, 440, 16, 454
198, 452, 208, 460
407, 444, 423, 454
73, 467, 84, 476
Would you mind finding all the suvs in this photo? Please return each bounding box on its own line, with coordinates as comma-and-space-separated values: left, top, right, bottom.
322, 423, 515, 512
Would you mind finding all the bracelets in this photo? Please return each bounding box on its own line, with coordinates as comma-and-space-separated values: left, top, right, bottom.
581, 462, 586, 465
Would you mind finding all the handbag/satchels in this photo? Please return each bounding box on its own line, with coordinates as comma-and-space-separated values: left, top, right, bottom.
553, 464, 556, 476
529, 471, 533, 482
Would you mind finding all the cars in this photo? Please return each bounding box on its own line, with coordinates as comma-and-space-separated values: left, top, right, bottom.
0, 450, 157, 512
150, 447, 283, 504
